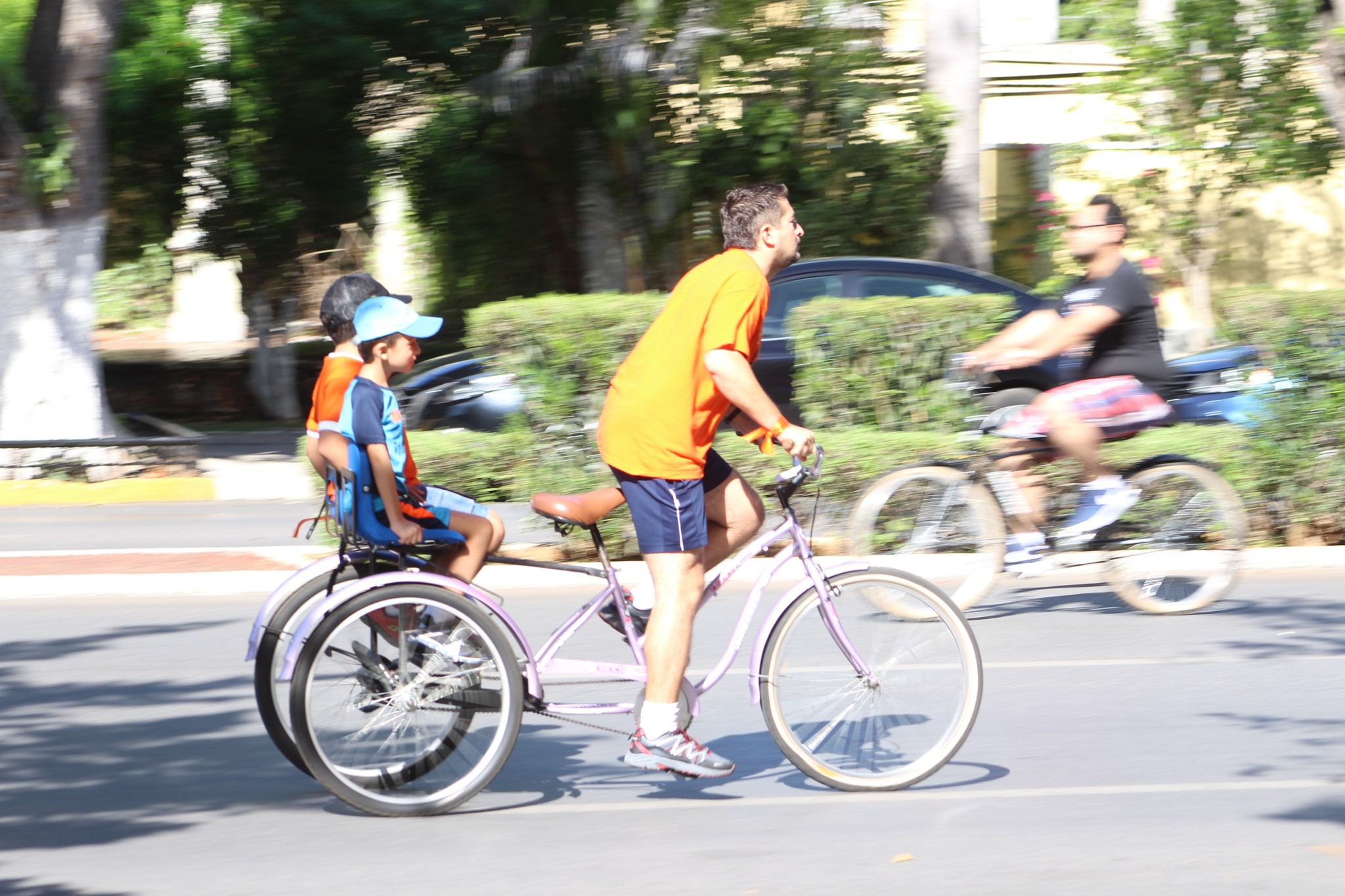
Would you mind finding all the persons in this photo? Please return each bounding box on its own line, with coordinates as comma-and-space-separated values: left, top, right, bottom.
962, 191, 1175, 569
309, 274, 506, 664
595, 182, 816, 778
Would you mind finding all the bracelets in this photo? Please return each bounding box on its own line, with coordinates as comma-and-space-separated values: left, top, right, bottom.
735, 413, 788, 455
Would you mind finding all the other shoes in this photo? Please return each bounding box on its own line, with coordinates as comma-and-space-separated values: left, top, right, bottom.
999, 532, 1047, 566
1054, 477, 1140, 536
360, 604, 418, 648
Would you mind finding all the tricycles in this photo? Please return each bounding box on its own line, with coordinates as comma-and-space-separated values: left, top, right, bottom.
241, 423, 988, 823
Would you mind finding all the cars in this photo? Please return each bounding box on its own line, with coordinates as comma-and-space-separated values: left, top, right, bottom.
724, 253, 1305, 439
387, 337, 620, 429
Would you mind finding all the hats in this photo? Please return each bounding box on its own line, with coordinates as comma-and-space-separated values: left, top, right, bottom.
320, 272, 413, 329
350, 295, 444, 346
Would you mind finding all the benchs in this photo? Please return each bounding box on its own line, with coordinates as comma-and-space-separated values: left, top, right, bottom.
315, 434, 465, 552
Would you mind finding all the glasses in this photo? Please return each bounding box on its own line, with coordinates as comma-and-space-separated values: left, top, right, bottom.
1067, 222, 1114, 237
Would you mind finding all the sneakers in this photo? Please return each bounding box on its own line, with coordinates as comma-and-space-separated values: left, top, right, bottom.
624, 724, 737, 779
598, 587, 653, 638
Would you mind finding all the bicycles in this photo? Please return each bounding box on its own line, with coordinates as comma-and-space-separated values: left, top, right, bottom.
840, 337, 1277, 625
